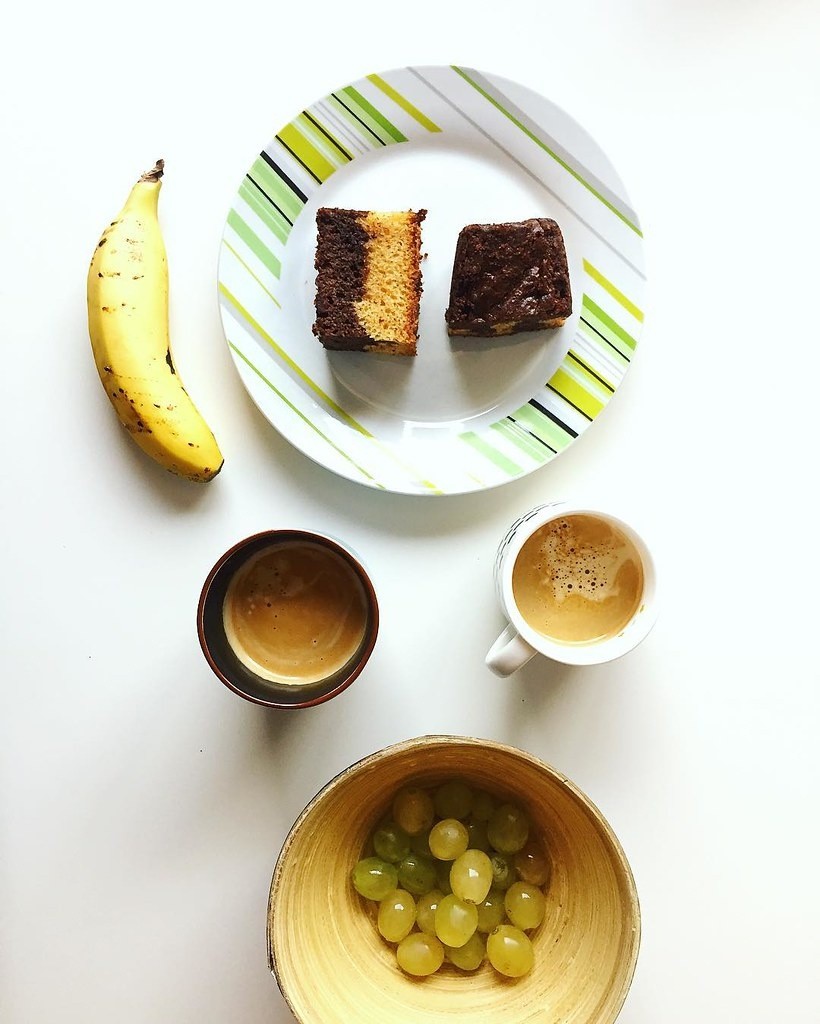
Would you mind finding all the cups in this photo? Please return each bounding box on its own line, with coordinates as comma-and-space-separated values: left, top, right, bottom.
196, 528, 380, 711
483, 500, 664, 678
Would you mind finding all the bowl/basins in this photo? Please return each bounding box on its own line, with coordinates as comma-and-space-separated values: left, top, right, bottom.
267, 735, 641, 1023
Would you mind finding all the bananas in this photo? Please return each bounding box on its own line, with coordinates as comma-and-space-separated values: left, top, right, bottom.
86, 159, 224, 484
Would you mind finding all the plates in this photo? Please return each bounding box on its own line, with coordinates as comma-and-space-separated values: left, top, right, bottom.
219, 64, 645, 496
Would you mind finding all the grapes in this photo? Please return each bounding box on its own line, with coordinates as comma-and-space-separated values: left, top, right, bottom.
352, 779, 548, 978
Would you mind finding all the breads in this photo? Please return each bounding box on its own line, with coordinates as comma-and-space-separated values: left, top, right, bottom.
444, 218, 572, 339
312, 207, 429, 355
